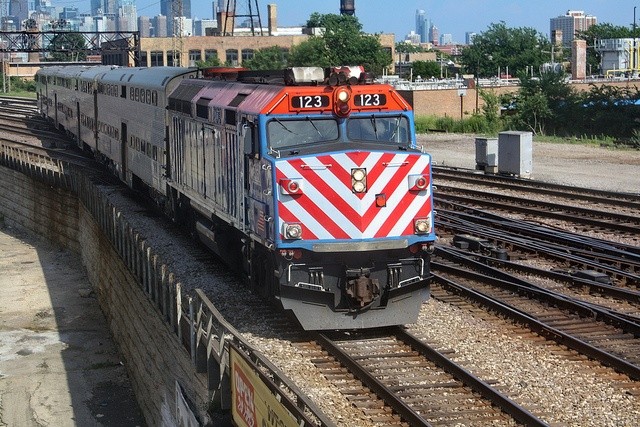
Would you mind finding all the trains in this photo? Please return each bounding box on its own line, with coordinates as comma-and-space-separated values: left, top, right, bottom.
35, 65, 436, 331
